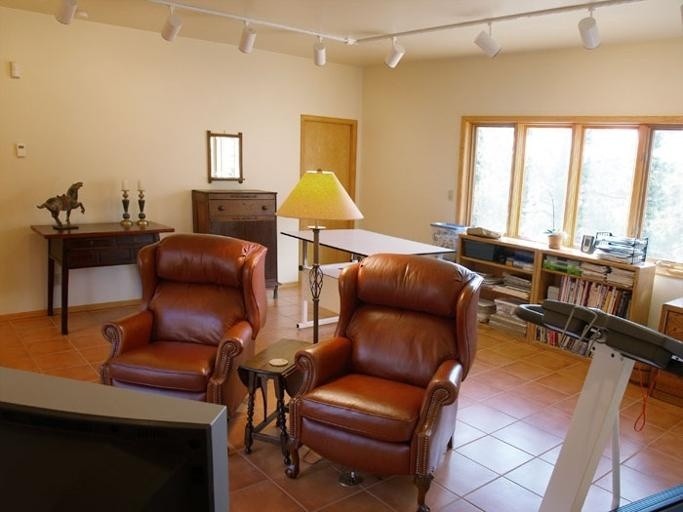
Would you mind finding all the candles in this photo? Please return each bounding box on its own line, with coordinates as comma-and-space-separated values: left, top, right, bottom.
121, 179, 128, 190
137, 180, 144, 190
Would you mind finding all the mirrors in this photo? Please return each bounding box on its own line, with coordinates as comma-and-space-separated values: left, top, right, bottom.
206, 130, 245, 184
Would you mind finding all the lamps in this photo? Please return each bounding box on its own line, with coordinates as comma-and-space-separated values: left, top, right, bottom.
271, 166, 363, 344
51, 0, 605, 68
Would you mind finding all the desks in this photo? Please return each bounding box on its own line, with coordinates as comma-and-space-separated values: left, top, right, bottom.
31, 217, 173, 332
237, 336, 315, 465
275, 224, 458, 331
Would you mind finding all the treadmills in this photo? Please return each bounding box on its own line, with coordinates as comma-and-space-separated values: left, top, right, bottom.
515, 302, 683, 512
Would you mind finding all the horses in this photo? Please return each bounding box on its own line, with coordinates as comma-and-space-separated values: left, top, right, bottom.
37, 182, 85, 226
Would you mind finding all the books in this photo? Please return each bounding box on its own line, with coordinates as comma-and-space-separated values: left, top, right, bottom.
476, 263, 635, 358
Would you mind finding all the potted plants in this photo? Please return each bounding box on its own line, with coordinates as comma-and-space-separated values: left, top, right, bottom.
543, 227, 563, 249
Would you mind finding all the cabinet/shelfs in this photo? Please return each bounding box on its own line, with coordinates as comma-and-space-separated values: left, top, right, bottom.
452, 233, 539, 343
647, 294, 681, 414
529, 242, 654, 362
192, 190, 282, 297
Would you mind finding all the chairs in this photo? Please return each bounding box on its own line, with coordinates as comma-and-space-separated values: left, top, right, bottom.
291, 256, 482, 511
98, 231, 268, 425
